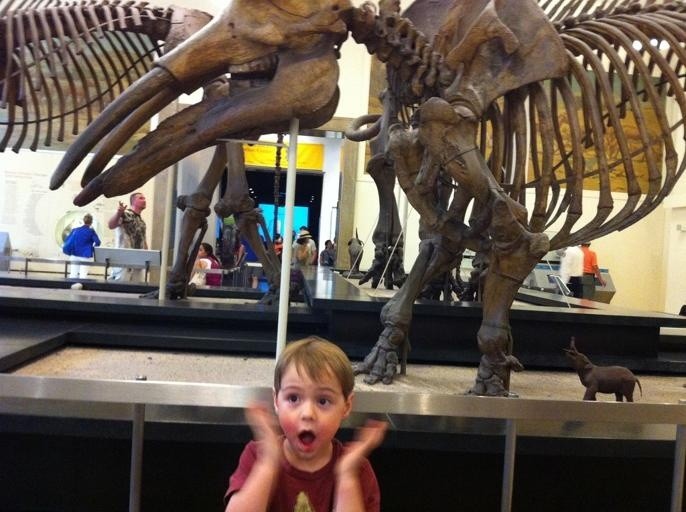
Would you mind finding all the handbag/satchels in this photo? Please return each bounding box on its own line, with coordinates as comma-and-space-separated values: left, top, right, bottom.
206, 263, 221, 286
63, 238, 73, 256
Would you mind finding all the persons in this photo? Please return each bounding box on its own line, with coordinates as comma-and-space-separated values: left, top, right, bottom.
108, 193, 148, 283
70, 213, 100, 279
223, 335, 389, 512
579, 244, 606, 300
216, 225, 333, 288
188, 244, 213, 286
559, 246, 584, 298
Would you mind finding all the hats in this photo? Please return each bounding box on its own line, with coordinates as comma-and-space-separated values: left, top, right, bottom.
584, 241, 592, 245
300, 230, 312, 238
